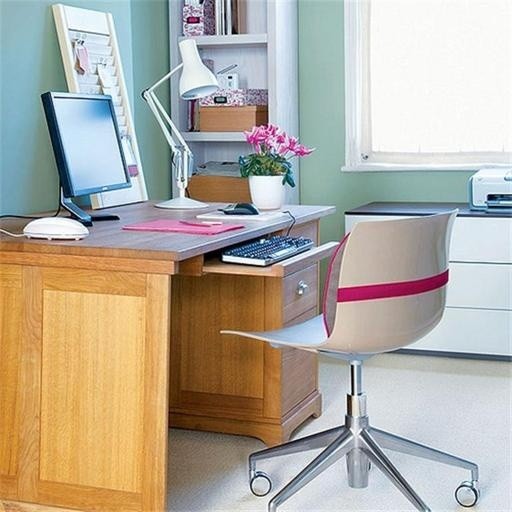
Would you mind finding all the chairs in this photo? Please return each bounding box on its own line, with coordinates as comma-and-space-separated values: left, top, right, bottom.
220, 207, 482, 511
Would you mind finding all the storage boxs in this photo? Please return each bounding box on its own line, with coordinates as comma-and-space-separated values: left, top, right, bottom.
187, 174, 250, 204
199, 106, 266, 134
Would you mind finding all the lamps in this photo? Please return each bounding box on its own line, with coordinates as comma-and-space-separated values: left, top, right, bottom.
142, 38, 218, 209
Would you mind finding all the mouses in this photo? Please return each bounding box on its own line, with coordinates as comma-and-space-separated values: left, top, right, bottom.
225, 203, 257, 215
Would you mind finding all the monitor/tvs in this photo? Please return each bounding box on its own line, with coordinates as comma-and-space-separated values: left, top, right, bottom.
40, 90, 132, 226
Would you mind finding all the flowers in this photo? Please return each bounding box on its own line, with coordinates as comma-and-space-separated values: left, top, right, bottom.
238, 123, 316, 188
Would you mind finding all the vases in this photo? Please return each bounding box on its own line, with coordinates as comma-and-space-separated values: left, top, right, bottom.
250, 174, 285, 209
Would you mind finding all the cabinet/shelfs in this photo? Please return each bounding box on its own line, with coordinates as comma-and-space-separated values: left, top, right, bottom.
344, 215, 511, 362
166, 0, 299, 208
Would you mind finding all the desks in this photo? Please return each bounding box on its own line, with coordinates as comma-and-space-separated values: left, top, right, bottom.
0, 200, 339, 510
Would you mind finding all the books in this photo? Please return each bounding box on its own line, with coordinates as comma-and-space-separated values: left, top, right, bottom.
182, 1, 239, 38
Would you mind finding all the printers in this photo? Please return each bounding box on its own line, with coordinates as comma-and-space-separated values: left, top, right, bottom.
469, 168, 512, 214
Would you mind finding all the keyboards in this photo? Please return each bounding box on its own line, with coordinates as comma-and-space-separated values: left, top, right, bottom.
220, 236, 313, 267
